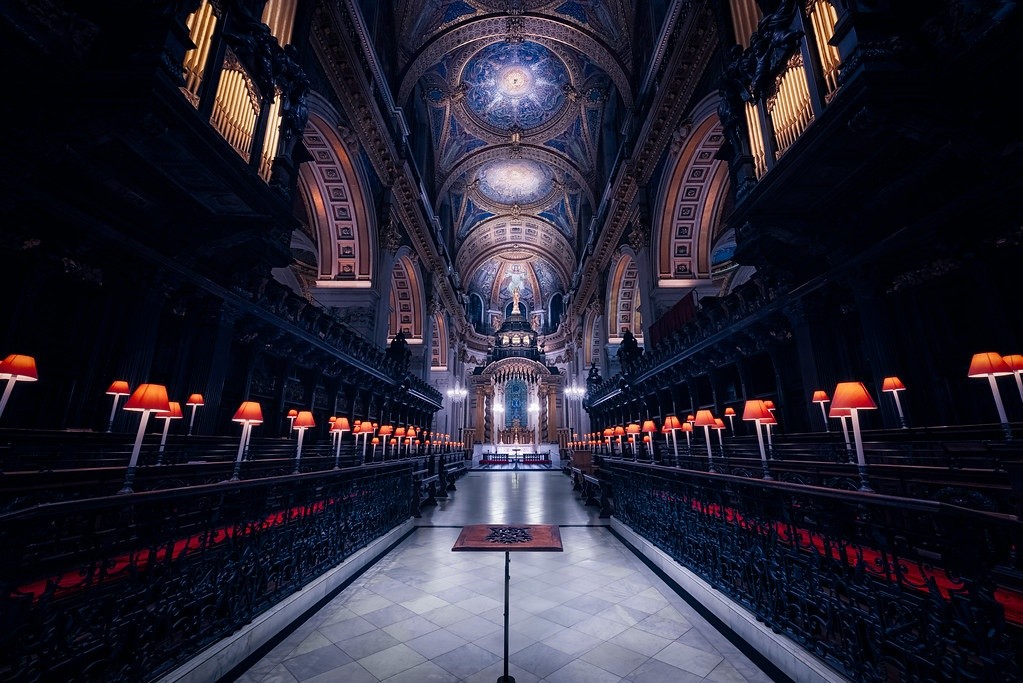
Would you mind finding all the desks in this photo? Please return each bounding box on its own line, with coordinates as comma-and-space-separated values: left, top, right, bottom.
451, 523, 564, 683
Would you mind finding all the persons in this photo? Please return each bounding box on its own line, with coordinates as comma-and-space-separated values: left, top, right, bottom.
511, 287, 521, 315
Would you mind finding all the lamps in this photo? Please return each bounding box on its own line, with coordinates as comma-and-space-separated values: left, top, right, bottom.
0, 351, 1023, 470
